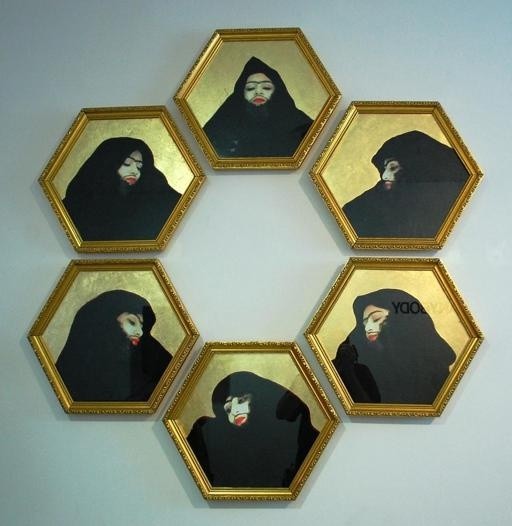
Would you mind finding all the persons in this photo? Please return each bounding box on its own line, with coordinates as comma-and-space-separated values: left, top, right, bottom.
55, 289, 174, 402
187, 370, 319, 487
63, 136, 181, 241
340, 130, 471, 237
202, 55, 314, 156
332, 289, 456, 404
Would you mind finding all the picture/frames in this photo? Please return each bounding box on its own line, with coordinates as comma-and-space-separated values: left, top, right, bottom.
308, 99, 484, 250
26, 258, 200, 415
173, 27, 342, 171
161, 341, 341, 500
302, 257, 484, 417
37, 103, 207, 254
